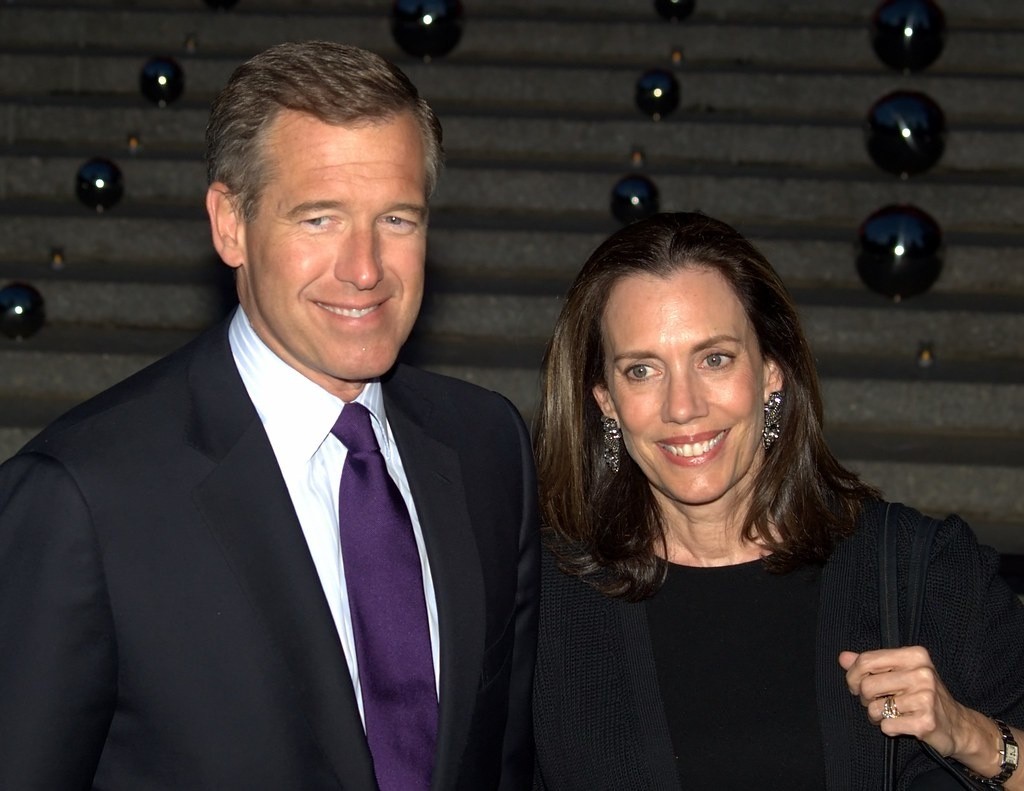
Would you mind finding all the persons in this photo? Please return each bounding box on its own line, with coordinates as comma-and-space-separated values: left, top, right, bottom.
0, 40, 545, 791
529, 204, 1024, 791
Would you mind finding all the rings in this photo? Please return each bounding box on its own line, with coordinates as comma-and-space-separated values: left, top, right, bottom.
881, 696, 900, 719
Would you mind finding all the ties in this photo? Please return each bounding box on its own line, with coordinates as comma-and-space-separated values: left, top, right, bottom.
328, 399, 442, 790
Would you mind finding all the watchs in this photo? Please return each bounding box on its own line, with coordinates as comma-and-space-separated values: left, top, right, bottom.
964, 716, 1019, 786
